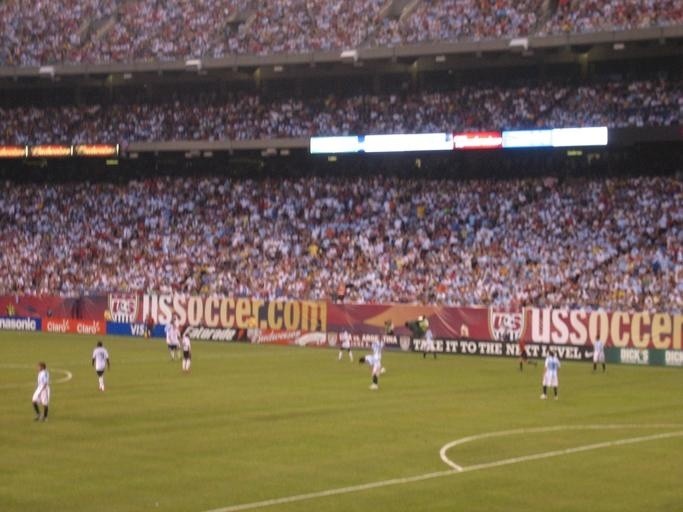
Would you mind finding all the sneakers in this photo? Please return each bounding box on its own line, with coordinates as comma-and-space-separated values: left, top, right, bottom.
370, 382, 380, 392
34, 414, 48, 423
539, 393, 559, 401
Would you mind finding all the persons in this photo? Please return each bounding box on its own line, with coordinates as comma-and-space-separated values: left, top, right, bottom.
592, 334, 606, 373
458, 318, 472, 338
164, 317, 193, 371
0, 0, 683, 313
517, 334, 537, 371
30, 361, 51, 422
334, 316, 438, 390
91, 341, 111, 391
540, 350, 561, 400
